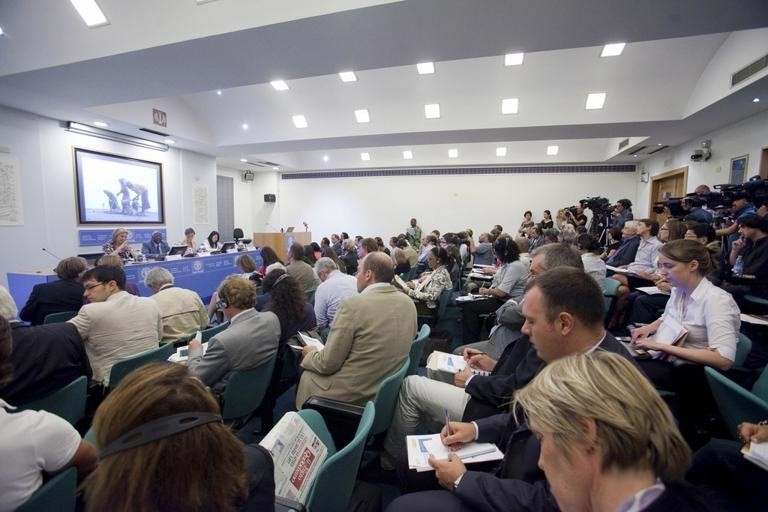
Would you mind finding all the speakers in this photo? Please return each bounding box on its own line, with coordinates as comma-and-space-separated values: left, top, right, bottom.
245, 173, 254, 180
264, 194, 275, 202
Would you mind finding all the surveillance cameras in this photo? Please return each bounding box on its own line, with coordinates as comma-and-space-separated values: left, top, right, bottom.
691, 150, 705, 162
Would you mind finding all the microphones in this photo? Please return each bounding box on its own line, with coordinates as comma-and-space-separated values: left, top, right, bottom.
303, 222, 308, 229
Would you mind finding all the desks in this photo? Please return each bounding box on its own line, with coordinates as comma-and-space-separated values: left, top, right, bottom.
6, 248, 263, 324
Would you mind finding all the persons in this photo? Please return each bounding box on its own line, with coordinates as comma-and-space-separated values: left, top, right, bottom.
125, 181, 151, 214
131, 196, 141, 214
142, 230, 170, 259
1, 320, 97, 511
0, 254, 211, 402
103, 190, 120, 212
166, 175, 768, 512
83, 359, 251, 512
103, 229, 140, 264
116, 178, 133, 216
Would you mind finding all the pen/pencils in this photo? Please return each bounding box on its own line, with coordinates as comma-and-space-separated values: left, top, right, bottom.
481, 281, 486, 287
445, 409, 449, 432
459, 352, 487, 355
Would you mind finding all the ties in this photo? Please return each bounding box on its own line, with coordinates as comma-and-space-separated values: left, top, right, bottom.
157, 244, 160, 254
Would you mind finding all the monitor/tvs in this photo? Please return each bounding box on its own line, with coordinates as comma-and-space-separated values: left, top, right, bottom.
220, 243, 235, 253
286, 227, 295, 232
169, 246, 187, 256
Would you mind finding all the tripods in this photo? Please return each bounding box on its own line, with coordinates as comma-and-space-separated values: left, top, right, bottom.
598, 213, 610, 246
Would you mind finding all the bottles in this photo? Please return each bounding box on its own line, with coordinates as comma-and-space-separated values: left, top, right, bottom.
734, 255, 744, 277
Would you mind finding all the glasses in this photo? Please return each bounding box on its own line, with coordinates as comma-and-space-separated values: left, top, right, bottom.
83, 281, 108, 292
657, 225, 669, 232
